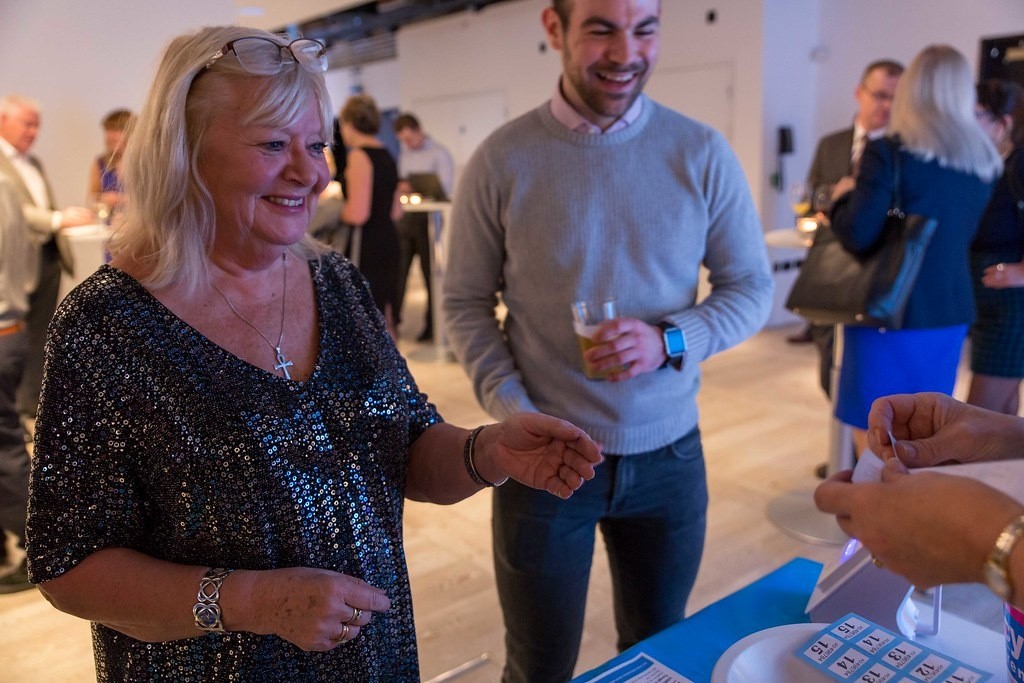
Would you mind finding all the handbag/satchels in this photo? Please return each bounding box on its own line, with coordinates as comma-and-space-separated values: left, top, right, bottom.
784, 133, 941, 333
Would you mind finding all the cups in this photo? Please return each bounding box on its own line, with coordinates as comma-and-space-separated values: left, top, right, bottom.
571, 295, 627, 380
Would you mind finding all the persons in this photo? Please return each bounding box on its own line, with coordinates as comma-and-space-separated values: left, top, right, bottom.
338, 96, 401, 316
0, 96, 89, 444
831, 45, 1003, 459
443, 1, 776, 683
24, 23, 604, 683
0, 171, 39, 594
389, 113, 455, 343
87, 110, 139, 263
967, 78, 1024, 414
813, 392, 1024, 611
799, 59, 904, 477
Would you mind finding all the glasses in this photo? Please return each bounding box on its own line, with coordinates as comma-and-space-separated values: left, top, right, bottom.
864, 84, 894, 103
192, 37, 329, 83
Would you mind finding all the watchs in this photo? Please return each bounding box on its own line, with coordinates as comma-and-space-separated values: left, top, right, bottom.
983, 515, 1024, 602
655, 319, 687, 370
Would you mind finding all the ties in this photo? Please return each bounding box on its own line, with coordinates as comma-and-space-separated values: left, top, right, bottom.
853, 135, 868, 170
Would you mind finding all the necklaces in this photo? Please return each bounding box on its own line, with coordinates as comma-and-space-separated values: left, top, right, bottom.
210, 250, 293, 382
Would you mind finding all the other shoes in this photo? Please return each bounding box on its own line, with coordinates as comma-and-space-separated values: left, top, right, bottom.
0, 558, 35, 595
416, 330, 433, 341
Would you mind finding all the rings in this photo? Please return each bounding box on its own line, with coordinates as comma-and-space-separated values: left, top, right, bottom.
871, 556, 881, 568
346, 607, 362, 625
337, 625, 349, 644
997, 263, 1004, 271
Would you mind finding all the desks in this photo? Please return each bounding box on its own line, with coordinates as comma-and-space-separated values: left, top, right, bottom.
53, 221, 111, 310
767, 229, 854, 547
566, 557, 1010, 683
402, 202, 459, 364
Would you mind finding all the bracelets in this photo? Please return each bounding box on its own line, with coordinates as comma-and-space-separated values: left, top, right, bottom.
463, 424, 509, 488
193, 568, 234, 636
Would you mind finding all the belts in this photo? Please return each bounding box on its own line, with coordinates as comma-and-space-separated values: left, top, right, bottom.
0, 319, 29, 336
